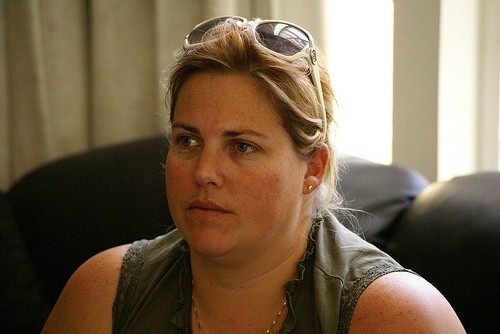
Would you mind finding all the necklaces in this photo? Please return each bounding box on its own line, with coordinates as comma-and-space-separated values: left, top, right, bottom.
191, 279, 287, 334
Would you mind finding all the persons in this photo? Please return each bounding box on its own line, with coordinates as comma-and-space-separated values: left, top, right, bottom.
37, 16, 468, 334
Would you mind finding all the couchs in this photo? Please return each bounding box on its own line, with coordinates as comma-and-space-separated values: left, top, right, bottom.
0, 134, 500, 334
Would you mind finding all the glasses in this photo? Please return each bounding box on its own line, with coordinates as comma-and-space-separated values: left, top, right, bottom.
183, 16, 327, 144
